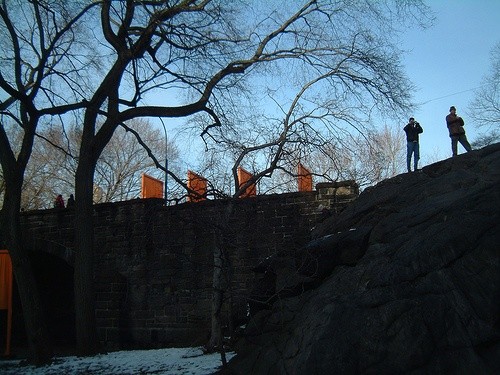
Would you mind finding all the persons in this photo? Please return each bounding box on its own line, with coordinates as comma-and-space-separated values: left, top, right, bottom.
66, 194, 75, 208
403, 118, 423, 172
55, 195, 64, 208
446, 106, 472, 157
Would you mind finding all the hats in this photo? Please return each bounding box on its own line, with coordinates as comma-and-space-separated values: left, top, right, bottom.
449, 106, 456, 112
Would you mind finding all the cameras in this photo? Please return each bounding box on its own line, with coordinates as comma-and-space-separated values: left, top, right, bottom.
413, 121, 417, 124
